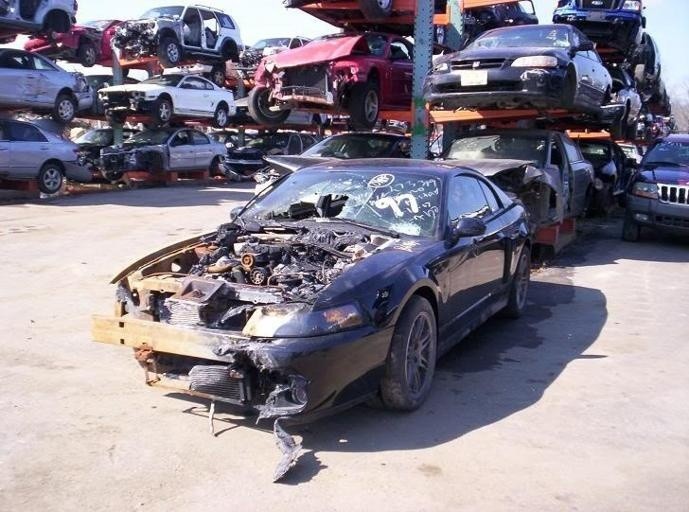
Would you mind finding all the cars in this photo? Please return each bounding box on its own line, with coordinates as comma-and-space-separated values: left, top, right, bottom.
92, 161, 533, 426
0, 0, 677, 234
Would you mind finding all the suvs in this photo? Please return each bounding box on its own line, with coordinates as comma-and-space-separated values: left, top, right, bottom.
620, 132, 689, 242
